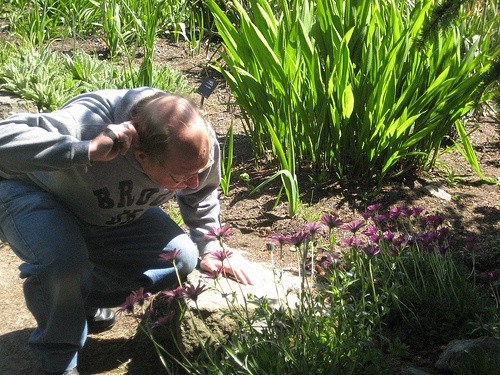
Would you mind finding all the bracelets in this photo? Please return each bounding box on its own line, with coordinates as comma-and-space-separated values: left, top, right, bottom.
101, 127, 122, 151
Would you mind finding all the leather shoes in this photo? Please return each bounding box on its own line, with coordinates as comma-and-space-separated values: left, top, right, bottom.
45, 368, 80, 375
85, 302, 115, 333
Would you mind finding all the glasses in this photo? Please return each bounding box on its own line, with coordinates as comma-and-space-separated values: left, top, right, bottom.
152, 154, 215, 183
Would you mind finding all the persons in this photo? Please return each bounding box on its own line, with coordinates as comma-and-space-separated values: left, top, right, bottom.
0, 86, 253, 375
